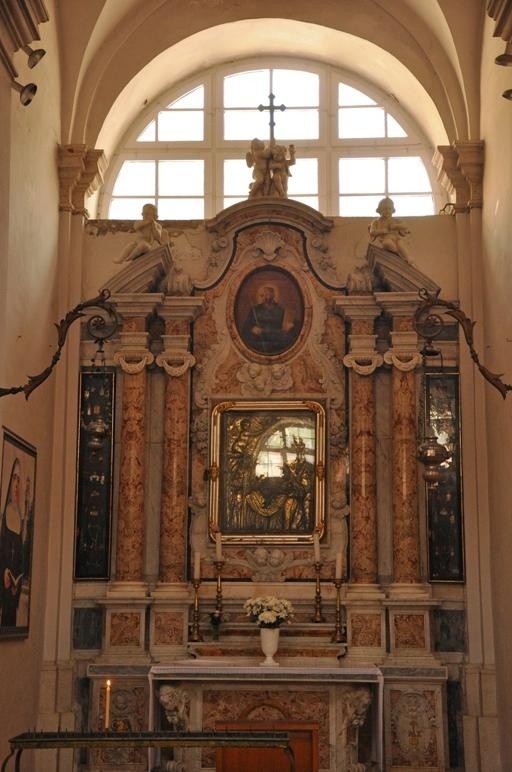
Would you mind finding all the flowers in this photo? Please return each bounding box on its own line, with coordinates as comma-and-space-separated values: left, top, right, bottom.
242, 593, 296, 628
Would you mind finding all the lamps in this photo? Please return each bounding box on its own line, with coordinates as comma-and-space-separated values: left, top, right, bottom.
11, 43, 46, 107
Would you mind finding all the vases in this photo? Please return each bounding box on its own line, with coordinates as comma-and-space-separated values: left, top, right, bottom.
258, 629, 280, 667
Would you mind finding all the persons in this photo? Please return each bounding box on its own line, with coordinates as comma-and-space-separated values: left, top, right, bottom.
368, 197, 416, 265
112, 202, 171, 266
1, 456, 34, 626
270, 141, 298, 199
242, 286, 296, 351
225, 417, 268, 458
244, 136, 274, 196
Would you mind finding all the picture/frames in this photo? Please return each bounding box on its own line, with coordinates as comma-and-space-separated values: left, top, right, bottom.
0, 422, 37, 642
208, 399, 329, 546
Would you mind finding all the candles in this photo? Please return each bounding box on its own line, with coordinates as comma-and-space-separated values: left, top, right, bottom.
104, 686, 110, 728
214, 532, 221, 559
192, 549, 201, 579
335, 551, 342, 578
313, 533, 320, 563
